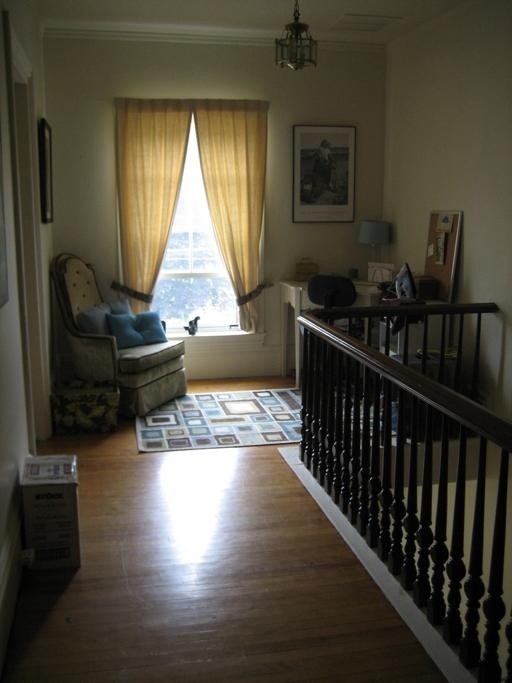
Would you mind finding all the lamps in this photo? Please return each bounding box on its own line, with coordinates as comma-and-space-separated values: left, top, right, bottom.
307, 274, 357, 323
51, 253, 188, 419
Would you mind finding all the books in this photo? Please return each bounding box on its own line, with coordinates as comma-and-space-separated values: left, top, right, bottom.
20, 453, 81, 572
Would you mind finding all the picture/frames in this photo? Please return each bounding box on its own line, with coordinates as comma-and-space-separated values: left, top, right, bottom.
279, 280, 383, 387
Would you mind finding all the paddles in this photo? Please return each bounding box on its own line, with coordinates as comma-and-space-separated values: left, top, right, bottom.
135, 388, 398, 452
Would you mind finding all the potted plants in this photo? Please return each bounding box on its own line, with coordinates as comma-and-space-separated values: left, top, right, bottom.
41, 117, 55, 225
291, 123, 357, 225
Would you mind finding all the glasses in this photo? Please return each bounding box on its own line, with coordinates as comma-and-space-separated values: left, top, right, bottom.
274, 1, 319, 72
358, 219, 391, 261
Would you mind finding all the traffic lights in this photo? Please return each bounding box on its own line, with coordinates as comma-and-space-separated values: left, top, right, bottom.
76, 298, 168, 350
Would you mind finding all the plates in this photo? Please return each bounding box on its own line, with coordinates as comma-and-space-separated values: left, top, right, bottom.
378, 300, 450, 382
